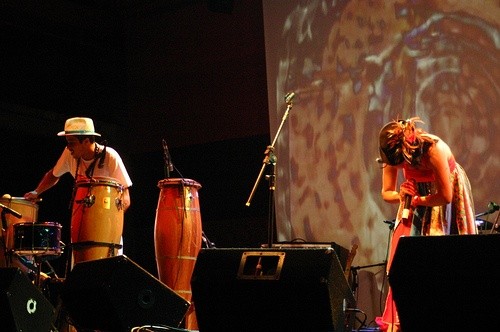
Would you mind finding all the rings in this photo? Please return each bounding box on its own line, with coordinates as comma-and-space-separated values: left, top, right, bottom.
406, 183, 409, 187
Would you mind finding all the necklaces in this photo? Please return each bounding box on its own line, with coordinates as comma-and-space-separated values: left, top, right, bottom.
82, 161, 88, 169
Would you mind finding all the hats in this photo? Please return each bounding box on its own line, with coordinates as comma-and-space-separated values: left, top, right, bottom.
57, 117, 101, 137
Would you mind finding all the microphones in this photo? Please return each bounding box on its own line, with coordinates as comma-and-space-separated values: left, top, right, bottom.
97, 143, 107, 169
162, 139, 173, 172
403, 178, 414, 219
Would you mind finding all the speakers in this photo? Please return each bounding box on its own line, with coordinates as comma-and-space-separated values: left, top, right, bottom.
66, 255, 191, 332
190, 248, 357, 332
0, 265, 53, 332
387, 233, 500, 332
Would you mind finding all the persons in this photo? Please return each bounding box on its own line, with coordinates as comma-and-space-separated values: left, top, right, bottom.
378, 117, 478, 332
24, 117, 133, 256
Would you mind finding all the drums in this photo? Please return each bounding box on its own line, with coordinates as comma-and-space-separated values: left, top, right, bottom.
0, 192, 38, 276
152, 176, 203, 331
12, 221, 62, 256
68, 174, 126, 332
